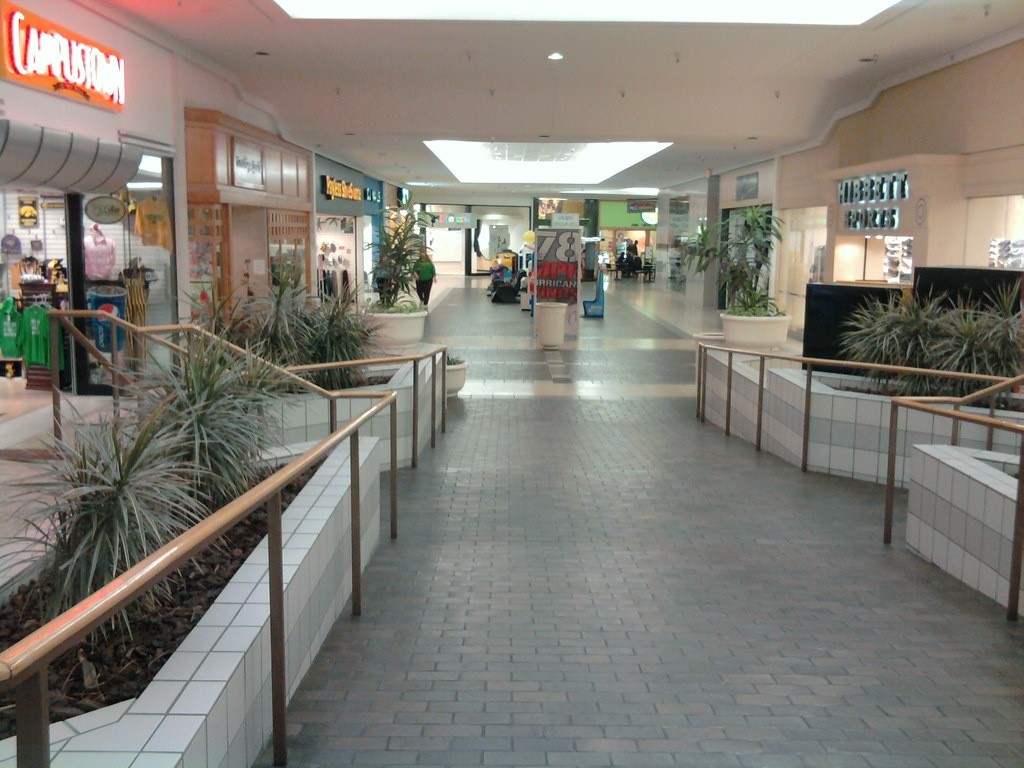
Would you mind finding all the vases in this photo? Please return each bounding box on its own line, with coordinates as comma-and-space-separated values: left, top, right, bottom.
534, 301, 568, 352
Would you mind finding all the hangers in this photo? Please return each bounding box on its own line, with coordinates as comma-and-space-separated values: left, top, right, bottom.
31, 293, 51, 309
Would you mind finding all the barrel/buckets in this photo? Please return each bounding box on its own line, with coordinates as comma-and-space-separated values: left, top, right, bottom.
535, 302, 569, 350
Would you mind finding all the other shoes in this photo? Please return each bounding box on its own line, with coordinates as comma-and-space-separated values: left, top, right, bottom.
419, 300, 424, 306
425, 305, 428, 308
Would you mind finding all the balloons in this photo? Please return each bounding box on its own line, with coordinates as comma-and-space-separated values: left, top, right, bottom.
524, 231, 536, 243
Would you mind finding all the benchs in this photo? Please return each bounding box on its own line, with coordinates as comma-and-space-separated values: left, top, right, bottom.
607, 264, 656, 283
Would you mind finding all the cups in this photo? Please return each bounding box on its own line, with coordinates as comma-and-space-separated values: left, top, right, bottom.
89, 286, 128, 353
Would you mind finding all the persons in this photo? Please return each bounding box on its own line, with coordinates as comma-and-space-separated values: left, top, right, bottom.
617, 240, 642, 278
414, 252, 437, 310
376, 254, 394, 305
117, 256, 158, 370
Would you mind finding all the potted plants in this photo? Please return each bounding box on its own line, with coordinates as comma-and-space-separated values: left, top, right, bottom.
357, 188, 430, 345
445, 354, 468, 398
672, 200, 793, 349
